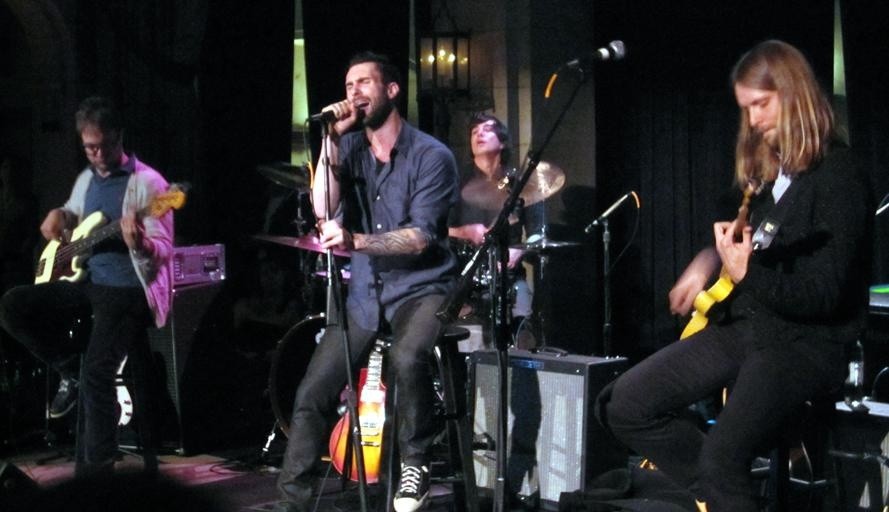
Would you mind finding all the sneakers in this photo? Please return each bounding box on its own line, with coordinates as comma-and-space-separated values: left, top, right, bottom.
393, 462, 432, 512
50, 376, 80, 418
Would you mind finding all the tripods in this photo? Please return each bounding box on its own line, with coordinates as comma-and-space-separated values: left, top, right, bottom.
311, 121, 369, 512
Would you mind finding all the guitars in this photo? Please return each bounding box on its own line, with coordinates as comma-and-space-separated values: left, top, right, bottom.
35, 183, 186, 284
329, 338, 387, 483
680, 179, 761, 339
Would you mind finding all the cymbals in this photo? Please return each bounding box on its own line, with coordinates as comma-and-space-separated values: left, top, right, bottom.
506, 243, 579, 249
253, 235, 352, 257
462, 159, 565, 210
249, 161, 312, 192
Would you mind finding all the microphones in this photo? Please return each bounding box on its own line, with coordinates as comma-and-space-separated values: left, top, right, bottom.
558, 39, 627, 74
584, 193, 630, 234
313, 103, 360, 121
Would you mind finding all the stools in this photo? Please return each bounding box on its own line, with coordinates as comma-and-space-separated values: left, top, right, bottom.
44, 310, 157, 477
339, 326, 481, 511
706, 382, 848, 511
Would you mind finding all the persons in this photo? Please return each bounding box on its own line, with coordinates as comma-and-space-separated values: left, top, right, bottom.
447, 113, 546, 318
274, 50, 459, 512
0, 97, 175, 504
594, 37, 873, 511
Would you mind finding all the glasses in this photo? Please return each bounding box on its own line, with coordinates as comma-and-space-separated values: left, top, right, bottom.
82, 143, 112, 156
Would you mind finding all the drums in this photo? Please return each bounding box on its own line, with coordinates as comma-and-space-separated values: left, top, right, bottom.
267, 313, 449, 462
